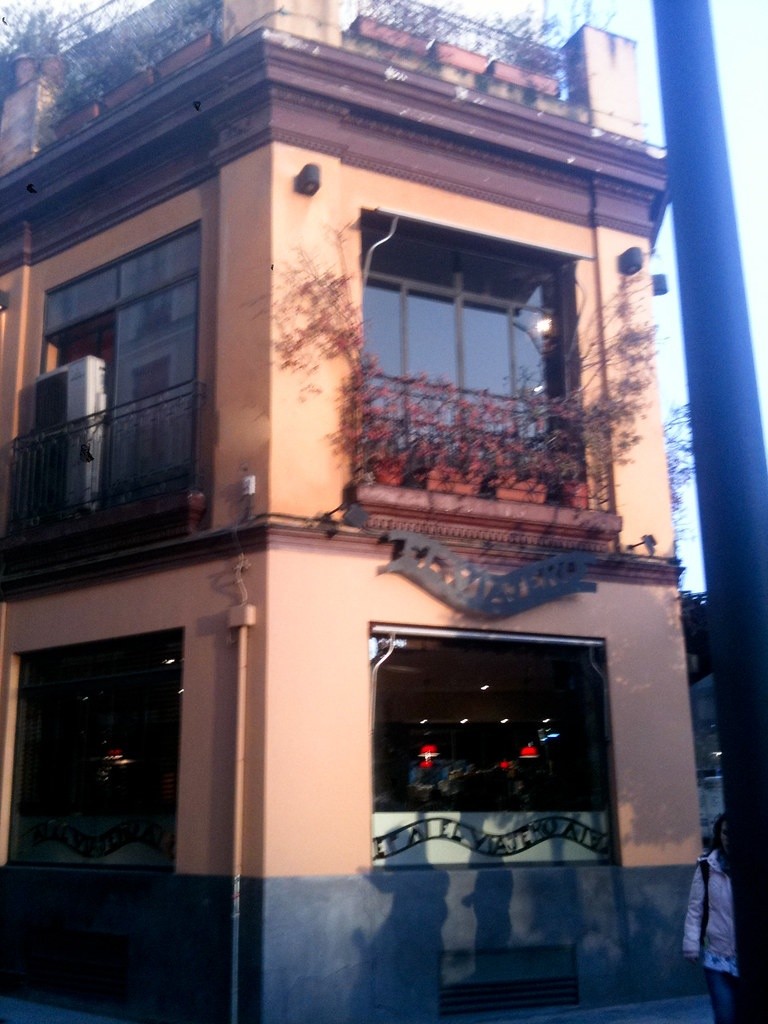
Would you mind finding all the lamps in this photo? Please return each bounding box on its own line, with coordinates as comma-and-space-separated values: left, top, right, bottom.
294, 163, 320, 197
620, 247, 643, 276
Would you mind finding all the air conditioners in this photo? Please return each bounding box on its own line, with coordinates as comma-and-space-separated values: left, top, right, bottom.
30, 355, 106, 513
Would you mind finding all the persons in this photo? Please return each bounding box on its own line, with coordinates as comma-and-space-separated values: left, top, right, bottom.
682, 812, 739, 1023
